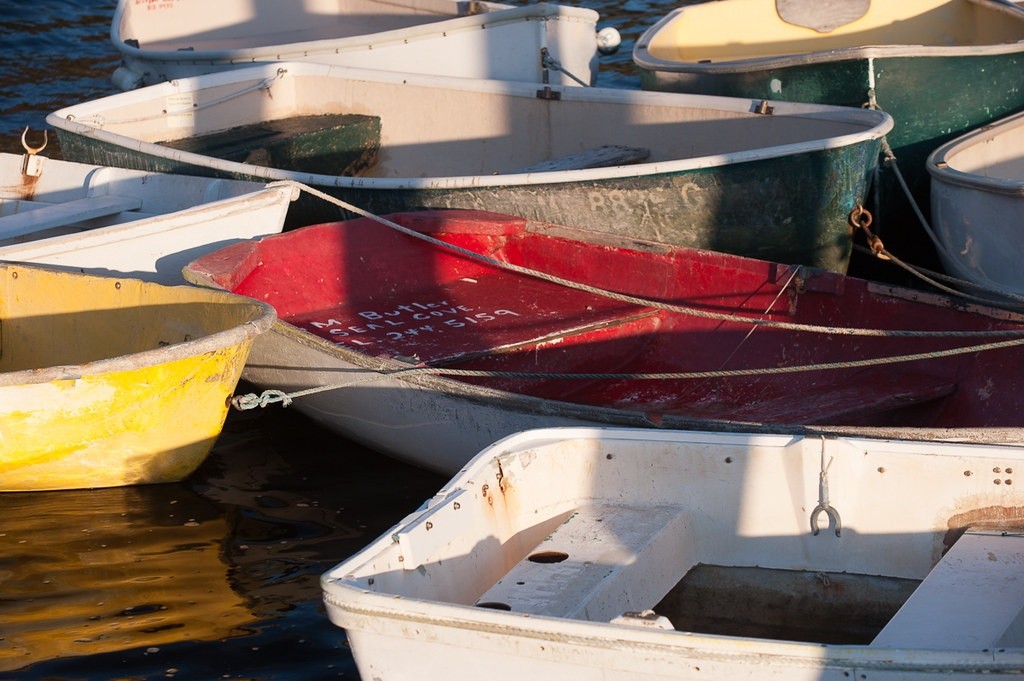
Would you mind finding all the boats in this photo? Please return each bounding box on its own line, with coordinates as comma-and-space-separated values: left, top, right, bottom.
0, 126, 304, 288
316, 422, 1024, 681
178, 204, 1024, 492
922, 108, 1024, 314
43, 60, 895, 277
629, 0, 1024, 189
103, 1, 621, 88
0, 259, 279, 494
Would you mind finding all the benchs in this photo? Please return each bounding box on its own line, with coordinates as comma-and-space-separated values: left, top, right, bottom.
159, 113, 381, 178
500, 144, 649, 175
307, 273, 661, 405
0, 194, 142, 242
476, 505, 696, 623
870, 527, 1024, 648
710, 369, 958, 426
